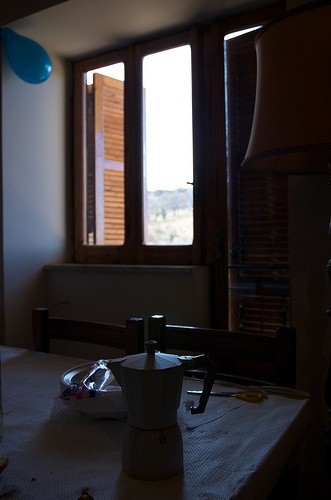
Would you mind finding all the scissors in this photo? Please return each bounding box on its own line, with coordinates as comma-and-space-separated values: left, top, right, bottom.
184, 385, 267, 404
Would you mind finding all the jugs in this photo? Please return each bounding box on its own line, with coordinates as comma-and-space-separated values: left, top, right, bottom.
107, 339, 218, 431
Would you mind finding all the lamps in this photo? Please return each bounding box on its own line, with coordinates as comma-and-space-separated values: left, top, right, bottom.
241, 0, 330, 425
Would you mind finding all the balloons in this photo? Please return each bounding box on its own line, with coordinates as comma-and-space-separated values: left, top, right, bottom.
1, 27, 52, 84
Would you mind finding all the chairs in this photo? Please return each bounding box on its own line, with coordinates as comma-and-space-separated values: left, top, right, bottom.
31, 308, 295, 391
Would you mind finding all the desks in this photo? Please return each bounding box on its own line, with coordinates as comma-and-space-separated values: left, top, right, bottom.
1, 345, 311, 500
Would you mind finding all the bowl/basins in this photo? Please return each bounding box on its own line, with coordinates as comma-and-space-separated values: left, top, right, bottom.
59, 360, 124, 419
